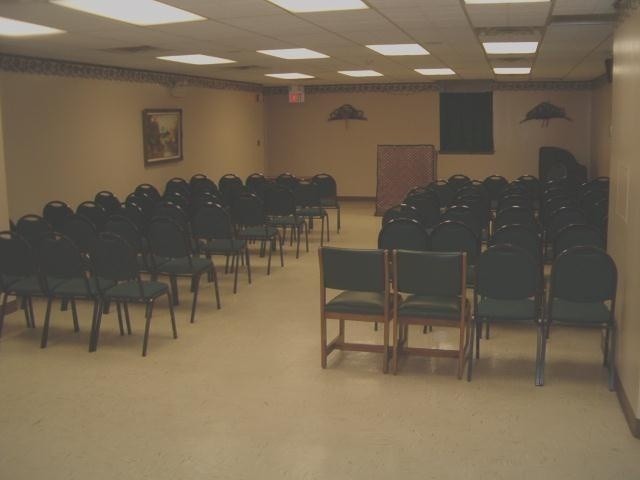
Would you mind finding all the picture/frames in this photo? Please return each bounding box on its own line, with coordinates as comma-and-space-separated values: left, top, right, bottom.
142, 105, 184, 165
